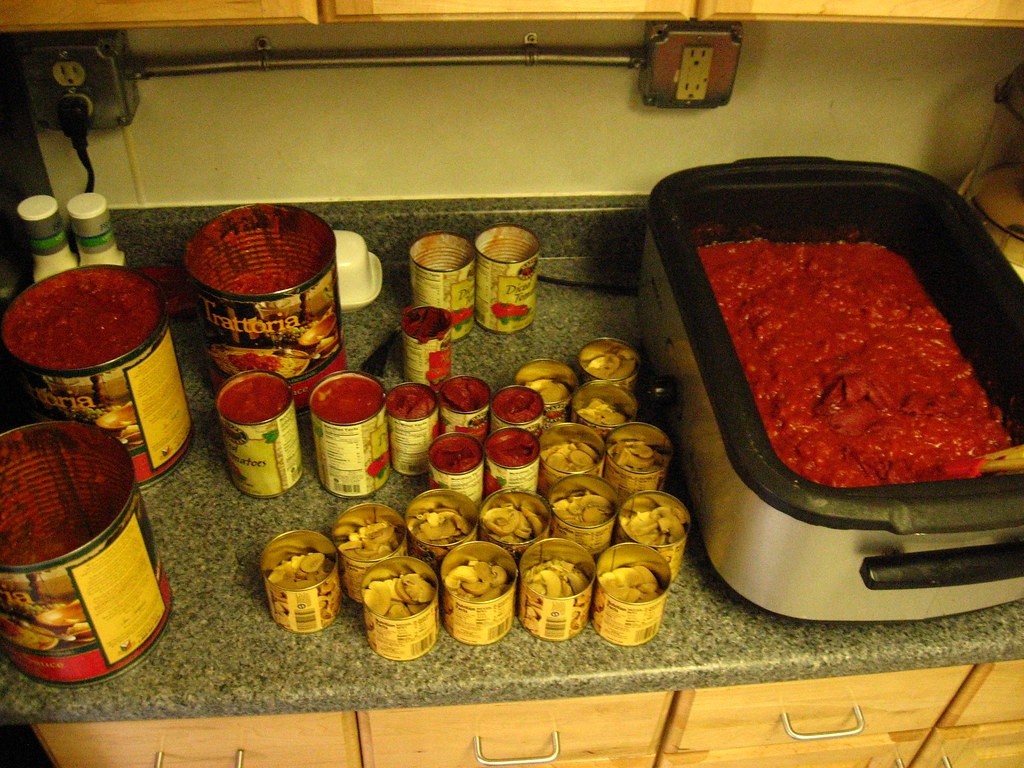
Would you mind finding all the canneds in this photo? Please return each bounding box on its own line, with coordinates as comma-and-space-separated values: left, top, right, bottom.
215, 223, 693, 662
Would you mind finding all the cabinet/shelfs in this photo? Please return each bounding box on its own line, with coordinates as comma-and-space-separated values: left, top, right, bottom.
33, 662, 1024, 768
0, 0, 1024, 31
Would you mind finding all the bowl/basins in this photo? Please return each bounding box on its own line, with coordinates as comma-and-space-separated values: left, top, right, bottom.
333, 229, 382, 313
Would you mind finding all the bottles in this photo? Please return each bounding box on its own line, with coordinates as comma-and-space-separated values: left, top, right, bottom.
66, 192, 127, 269
17, 194, 79, 285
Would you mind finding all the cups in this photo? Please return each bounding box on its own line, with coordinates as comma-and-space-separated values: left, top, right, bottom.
409, 233, 474, 345
474, 223, 542, 335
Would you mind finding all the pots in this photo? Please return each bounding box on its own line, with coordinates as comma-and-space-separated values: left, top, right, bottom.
637, 156, 1024, 620
972, 60, 1024, 242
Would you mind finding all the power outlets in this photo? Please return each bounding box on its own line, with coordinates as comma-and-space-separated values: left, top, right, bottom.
638, 22, 743, 108
18, 31, 140, 131
